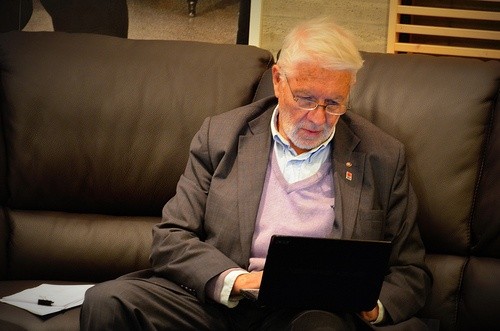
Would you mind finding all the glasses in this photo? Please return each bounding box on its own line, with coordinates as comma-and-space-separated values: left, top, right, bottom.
283, 72, 352, 115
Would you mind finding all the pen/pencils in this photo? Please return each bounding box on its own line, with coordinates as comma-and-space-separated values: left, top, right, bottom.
4, 297, 57, 306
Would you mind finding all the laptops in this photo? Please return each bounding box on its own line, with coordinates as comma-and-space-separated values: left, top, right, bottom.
241, 233, 392, 313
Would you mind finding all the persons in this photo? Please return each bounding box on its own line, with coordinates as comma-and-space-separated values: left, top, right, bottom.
81, 16, 433, 331
0, 1, 129, 39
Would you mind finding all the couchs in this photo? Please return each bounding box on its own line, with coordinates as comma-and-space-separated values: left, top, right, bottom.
1, 30, 500, 331
